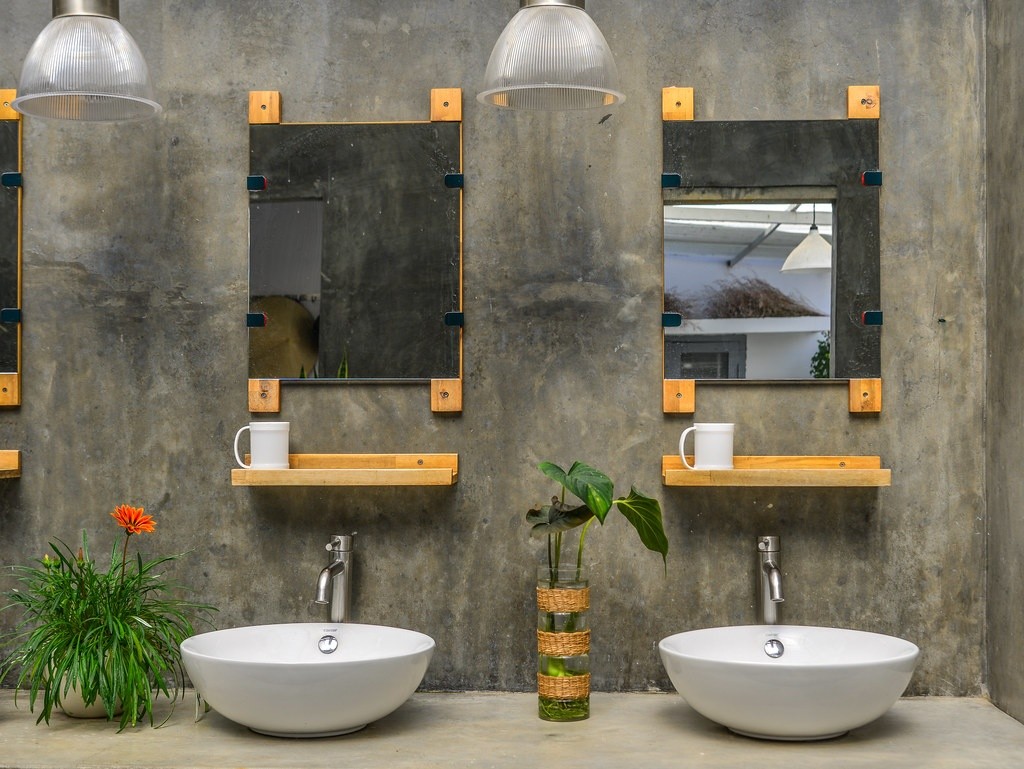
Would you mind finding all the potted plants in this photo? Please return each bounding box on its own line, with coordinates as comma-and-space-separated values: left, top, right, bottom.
527, 462, 667, 723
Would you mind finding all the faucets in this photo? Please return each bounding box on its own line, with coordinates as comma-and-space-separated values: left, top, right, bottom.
755, 535, 786, 626
314, 532, 355, 622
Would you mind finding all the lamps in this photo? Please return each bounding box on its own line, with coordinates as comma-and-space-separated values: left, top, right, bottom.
781, 202, 832, 270
9, 0, 163, 124
477, 0, 626, 112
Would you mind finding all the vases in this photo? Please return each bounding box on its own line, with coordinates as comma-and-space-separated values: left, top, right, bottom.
43, 649, 125, 718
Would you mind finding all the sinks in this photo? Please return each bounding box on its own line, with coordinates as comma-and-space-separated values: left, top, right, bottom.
180, 621, 436, 738
658, 624, 921, 741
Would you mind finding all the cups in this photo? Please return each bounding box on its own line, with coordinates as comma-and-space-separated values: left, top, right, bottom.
234, 421, 290, 469
679, 423, 737, 470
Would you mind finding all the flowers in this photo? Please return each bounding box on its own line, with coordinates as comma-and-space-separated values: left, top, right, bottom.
0, 504, 220, 734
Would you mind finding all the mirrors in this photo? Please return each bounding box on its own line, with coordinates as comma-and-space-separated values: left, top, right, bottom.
0, 118, 22, 373
660, 118, 881, 383
247, 121, 463, 385
